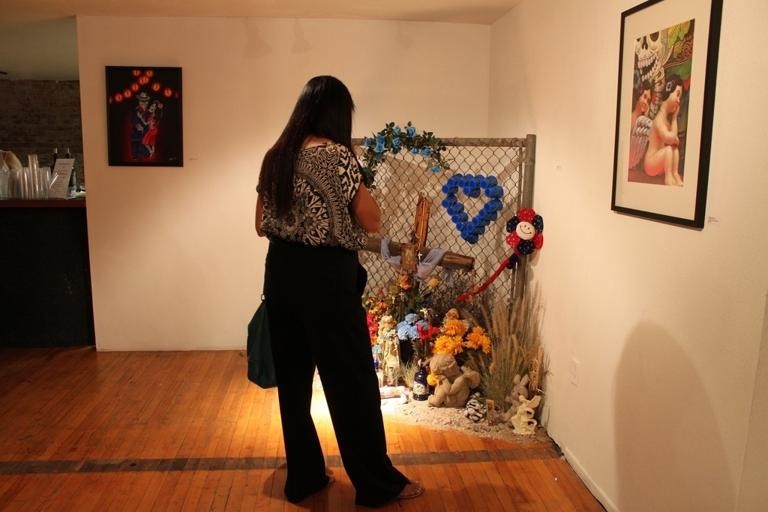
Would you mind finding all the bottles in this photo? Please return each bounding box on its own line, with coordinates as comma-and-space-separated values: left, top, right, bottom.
411, 358, 431, 402
65, 147, 77, 196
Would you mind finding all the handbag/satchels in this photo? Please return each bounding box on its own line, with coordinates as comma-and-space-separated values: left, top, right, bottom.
246, 301, 277, 388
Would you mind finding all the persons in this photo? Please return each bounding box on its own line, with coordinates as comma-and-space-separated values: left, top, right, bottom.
631, 74, 683, 186
254, 75, 424, 508
131, 90, 163, 160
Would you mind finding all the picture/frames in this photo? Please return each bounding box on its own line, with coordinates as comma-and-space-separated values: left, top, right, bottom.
104, 66, 183, 166
611, 0, 723, 230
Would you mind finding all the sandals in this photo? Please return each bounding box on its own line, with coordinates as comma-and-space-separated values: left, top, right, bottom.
324, 468, 335, 483
398, 480, 424, 498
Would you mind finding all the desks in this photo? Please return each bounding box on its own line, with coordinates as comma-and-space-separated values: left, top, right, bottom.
0, 197, 85, 209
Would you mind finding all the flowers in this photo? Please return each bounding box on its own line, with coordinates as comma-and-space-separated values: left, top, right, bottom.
359, 120, 491, 356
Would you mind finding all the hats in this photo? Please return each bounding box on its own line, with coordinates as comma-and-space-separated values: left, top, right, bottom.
136, 92, 150, 101
